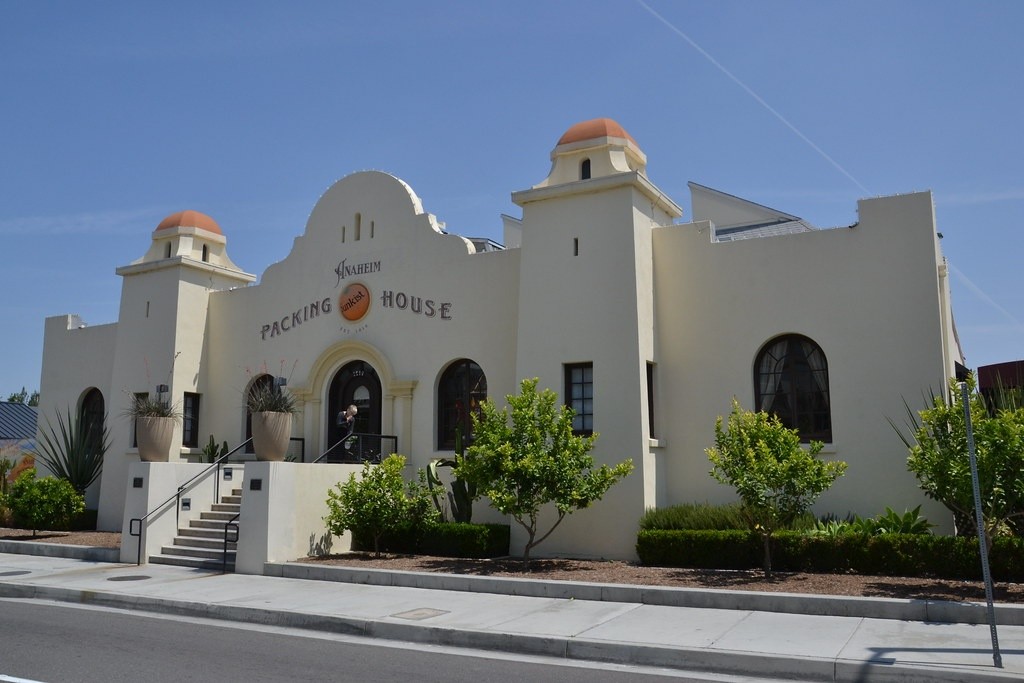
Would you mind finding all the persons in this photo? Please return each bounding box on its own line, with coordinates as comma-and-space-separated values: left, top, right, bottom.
336, 404, 358, 464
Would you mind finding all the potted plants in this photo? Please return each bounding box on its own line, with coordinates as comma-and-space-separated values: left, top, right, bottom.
237, 358, 305, 460
115, 351, 189, 463
